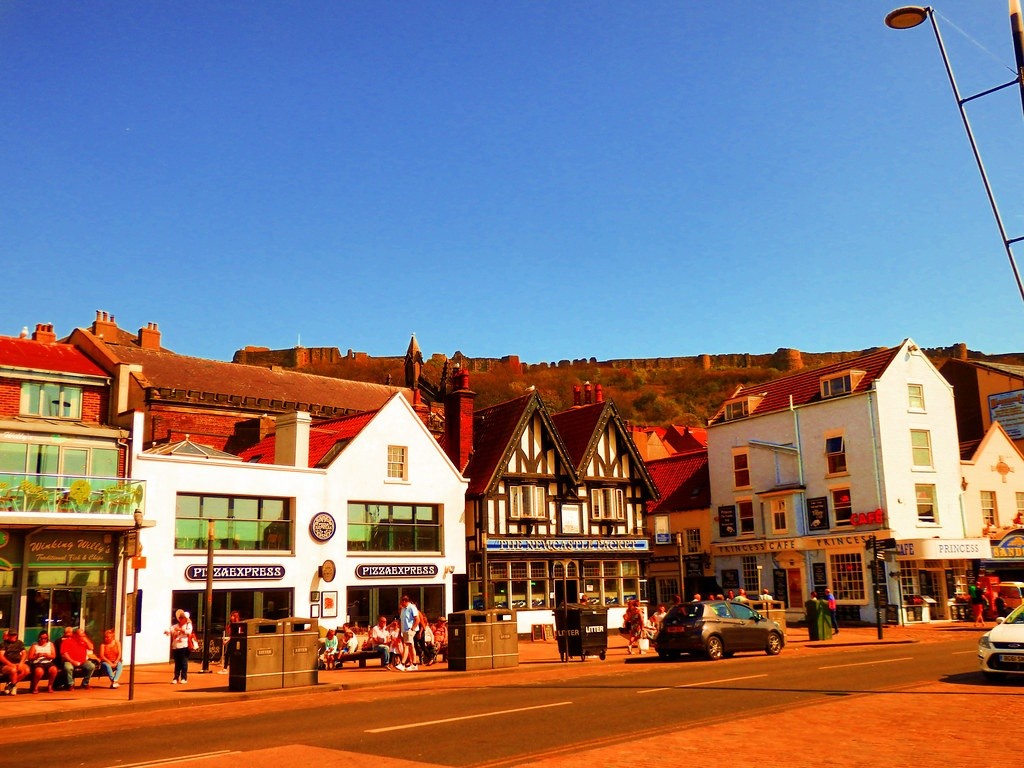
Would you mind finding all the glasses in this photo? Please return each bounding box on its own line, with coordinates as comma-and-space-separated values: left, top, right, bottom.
179, 617, 185, 619
9, 634, 17, 636
40, 638, 47, 640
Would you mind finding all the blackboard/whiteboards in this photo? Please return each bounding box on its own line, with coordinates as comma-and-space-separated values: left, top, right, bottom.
544, 624, 555, 641
532, 624, 544, 641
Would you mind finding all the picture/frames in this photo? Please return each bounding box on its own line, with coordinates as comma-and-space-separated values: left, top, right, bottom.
321, 591, 337, 618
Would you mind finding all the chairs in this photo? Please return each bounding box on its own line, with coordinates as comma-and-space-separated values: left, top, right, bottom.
0, 486, 137, 512
266, 532, 286, 550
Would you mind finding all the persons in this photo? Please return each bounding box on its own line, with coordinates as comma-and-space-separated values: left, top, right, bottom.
164, 609, 193, 684
623, 589, 774, 655
581, 595, 588, 605
323, 616, 447, 671
0, 626, 123, 696
811, 589, 840, 635
969, 582, 1008, 627
395, 595, 434, 672
217, 611, 241, 675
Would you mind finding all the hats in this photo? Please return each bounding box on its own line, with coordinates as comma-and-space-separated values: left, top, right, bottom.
438, 617, 447, 621
175, 609, 193, 624
8, 628, 18, 634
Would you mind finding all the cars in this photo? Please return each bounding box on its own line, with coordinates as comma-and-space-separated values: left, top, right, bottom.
654, 601, 786, 660
976, 602, 1024, 681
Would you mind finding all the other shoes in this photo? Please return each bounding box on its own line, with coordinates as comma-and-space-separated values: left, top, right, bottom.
406, 664, 418, 671
216, 669, 228, 675
5, 681, 119, 696
640, 650, 646, 654
171, 680, 179, 684
832, 630, 840, 636
180, 679, 187, 683
395, 663, 405, 672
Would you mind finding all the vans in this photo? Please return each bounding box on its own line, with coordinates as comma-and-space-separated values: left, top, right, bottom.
992, 580, 1024, 620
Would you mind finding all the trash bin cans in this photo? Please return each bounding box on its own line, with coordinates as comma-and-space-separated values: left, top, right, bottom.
805, 599, 832, 640
552, 601, 609, 661
228, 618, 283, 691
767, 600, 787, 637
279, 617, 319, 688
448, 610, 492, 671
742, 600, 767, 618
489, 609, 519, 669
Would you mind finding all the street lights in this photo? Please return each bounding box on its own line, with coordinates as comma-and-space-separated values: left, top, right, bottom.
127, 508, 144, 700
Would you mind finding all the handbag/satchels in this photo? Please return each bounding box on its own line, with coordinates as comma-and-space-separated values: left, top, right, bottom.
638, 639, 649, 651
186, 624, 199, 651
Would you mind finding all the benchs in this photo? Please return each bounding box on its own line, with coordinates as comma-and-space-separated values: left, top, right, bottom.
342, 644, 448, 666
0, 666, 117, 687
